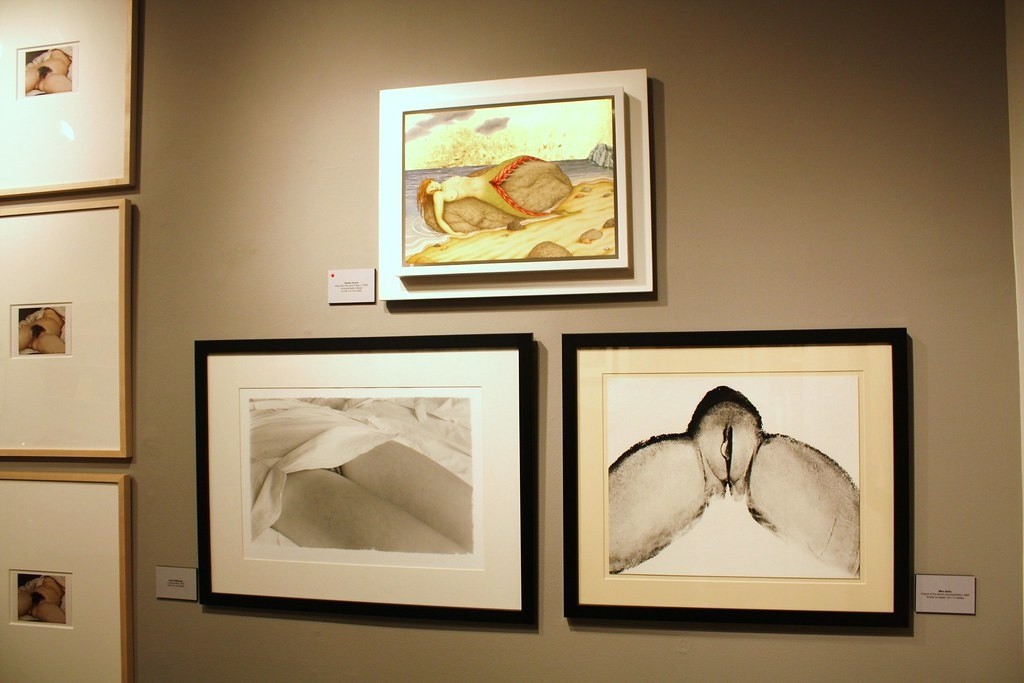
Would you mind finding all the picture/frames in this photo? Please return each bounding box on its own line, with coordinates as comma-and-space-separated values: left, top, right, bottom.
0, 0, 136, 198
563, 329, 911, 629
0, 199, 133, 458
194, 333, 535, 623
0, 472, 134, 683
377, 69, 653, 301
